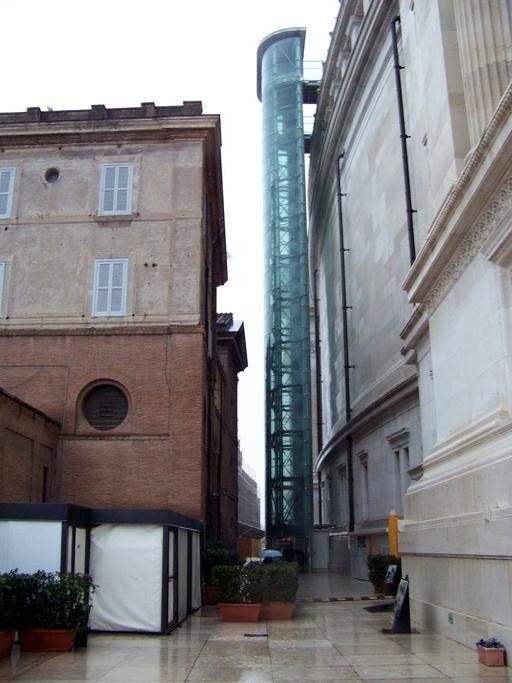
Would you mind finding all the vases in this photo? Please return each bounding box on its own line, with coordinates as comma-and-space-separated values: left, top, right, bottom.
476, 646, 504, 665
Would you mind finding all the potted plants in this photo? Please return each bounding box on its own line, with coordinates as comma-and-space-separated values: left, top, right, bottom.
265, 561, 298, 620
1, 572, 21, 659
365, 554, 402, 594
17, 567, 100, 653
209, 564, 263, 622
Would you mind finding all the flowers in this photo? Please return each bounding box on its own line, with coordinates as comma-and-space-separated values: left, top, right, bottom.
477, 637, 506, 649
